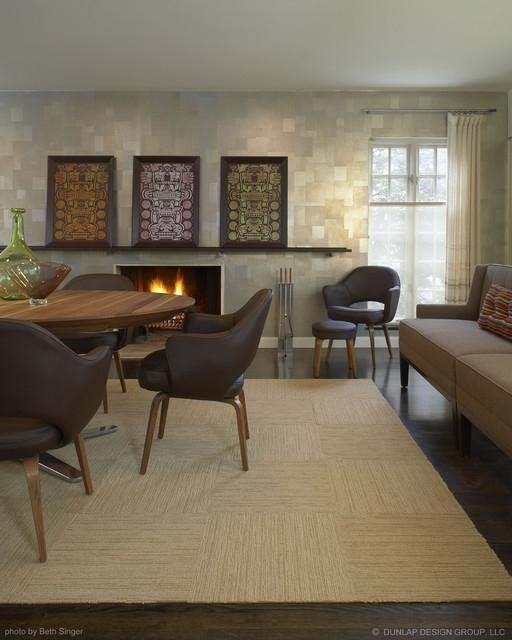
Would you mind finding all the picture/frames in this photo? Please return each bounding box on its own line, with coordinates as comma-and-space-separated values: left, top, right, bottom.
130, 154, 201, 248
44, 153, 118, 246
218, 155, 288, 249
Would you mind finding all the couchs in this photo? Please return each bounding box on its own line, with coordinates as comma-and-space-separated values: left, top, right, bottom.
398, 264, 512, 461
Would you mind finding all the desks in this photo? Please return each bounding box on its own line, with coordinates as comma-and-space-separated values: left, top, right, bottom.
1, 288, 196, 485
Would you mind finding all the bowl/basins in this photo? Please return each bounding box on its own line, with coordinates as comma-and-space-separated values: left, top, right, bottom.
4, 261, 72, 305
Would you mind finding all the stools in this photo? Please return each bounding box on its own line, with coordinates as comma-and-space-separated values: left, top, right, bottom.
311, 321, 357, 380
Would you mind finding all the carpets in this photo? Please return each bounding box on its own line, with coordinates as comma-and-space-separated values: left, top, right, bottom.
1, 379, 512, 603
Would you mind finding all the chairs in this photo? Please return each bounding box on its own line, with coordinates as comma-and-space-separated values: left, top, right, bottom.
0, 317, 113, 563
58, 273, 134, 414
138, 287, 274, 475
322, 265, 401, 369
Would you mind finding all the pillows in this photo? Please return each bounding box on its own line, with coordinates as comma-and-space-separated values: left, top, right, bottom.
476, 284, 511, 341
